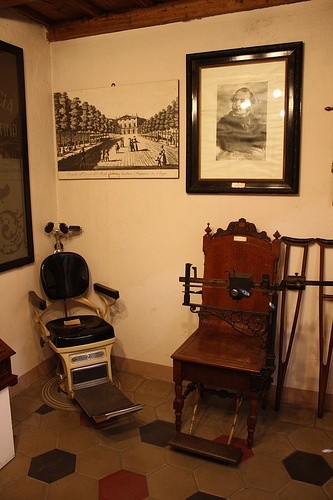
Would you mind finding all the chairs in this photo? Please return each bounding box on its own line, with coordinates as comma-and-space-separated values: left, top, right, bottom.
171, 218, 282, 465
27, 223, 145, 423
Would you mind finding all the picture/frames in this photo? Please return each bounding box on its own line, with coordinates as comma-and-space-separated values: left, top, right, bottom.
0, 40, 35, 271
186, 41, 305, 195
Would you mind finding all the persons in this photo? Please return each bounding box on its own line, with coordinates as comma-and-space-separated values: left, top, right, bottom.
81, 135, 174, 167
